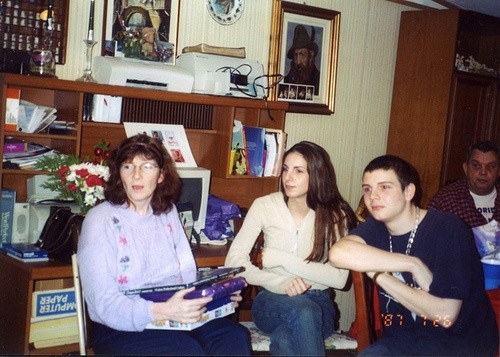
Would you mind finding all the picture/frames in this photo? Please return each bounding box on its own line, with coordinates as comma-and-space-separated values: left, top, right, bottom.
206, 0, 244, 25
268, 0, 342, 114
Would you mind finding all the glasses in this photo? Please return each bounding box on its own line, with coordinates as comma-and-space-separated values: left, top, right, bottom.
121, 162, 159, 174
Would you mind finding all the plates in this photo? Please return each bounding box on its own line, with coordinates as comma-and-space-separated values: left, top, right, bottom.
206, 0, 245, 25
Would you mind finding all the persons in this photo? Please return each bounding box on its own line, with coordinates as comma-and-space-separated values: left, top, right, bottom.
152, 131, 163, 145
424, 140, 500, 228
76, 132, 252, 357
278, 88, 312, 100
224, 141, 360, 357
174, 151, 185, 162
329, 154, 499, 357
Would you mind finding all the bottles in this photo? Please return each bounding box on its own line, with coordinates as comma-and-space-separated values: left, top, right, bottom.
27, 44, 56, 76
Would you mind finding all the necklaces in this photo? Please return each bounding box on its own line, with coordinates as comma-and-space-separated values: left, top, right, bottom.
389, 207, 420, 276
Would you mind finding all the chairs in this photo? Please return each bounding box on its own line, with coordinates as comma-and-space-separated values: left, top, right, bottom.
351, 269, 390, 350
232, 218, 360, 357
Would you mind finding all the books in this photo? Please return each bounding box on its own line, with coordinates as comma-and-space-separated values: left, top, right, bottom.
3, 138, 71, 172
4, 87, 57, 134
227, 119, 288, 177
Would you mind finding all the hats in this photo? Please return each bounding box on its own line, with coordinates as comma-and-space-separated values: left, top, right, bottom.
287, 24, 319, 59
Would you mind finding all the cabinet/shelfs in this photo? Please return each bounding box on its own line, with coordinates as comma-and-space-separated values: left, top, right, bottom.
387, 9, 500, 210
0, 68, 289, 357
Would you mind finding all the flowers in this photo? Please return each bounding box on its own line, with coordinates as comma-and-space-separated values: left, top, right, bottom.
34, 139, 115, 213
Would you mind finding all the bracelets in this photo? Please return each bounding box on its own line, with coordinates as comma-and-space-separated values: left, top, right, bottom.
373, 271, 386, 285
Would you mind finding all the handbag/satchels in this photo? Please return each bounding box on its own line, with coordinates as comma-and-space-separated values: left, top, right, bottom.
37, 206, 85, 264
